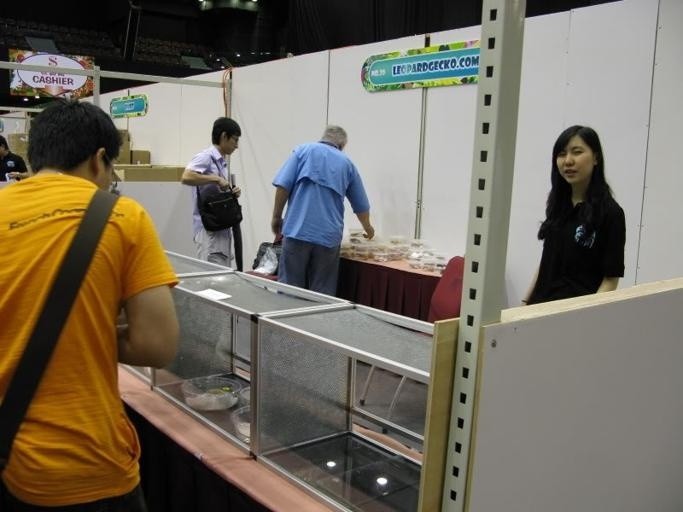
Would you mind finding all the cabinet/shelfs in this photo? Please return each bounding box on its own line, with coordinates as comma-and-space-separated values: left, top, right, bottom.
116, 248, 435, 512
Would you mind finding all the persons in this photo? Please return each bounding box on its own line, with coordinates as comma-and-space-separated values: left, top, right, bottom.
521, 125, 626, 306
180, 117, 240, 267
272, 125, 374, 296
0, 101, 181, 512
0, 135, 29, 182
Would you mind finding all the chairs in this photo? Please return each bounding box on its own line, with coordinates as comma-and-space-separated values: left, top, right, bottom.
358, 253, 465, 434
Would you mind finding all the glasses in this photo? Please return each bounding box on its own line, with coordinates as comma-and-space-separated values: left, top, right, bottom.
229, 133, 238, 142
105, 153, 118, 190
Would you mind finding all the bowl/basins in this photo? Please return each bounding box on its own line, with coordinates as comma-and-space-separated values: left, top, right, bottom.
239, 386, 253, 405
231, 406, 252, 444
181, 376, 241, 411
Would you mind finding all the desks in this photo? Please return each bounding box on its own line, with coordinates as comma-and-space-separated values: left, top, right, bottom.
340, 234, 451, 322
